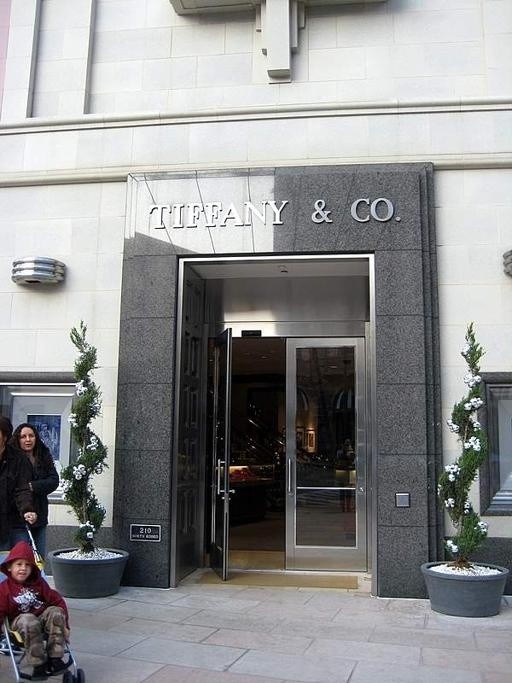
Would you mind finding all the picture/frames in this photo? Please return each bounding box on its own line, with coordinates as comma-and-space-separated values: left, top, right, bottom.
280, 425, 318, 457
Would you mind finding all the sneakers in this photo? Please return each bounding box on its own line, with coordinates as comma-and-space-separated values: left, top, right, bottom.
46, 657, 65, 674
19, 666, 48, 681
0, 634, 22, 655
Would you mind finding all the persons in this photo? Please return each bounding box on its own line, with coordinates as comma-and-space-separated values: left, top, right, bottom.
12, 423, 58, 572
338, 438, 355, 513
0, 417, 38, 551
0, 540, 69, 681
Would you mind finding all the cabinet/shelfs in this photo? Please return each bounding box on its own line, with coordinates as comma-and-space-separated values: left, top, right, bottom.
230, 461, 276, 526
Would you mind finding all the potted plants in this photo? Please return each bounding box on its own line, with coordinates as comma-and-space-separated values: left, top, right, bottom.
45, 320, 132, 599
421, 321, 510, 617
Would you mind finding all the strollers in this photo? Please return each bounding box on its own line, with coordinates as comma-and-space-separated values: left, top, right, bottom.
0, 522, 87, 683
